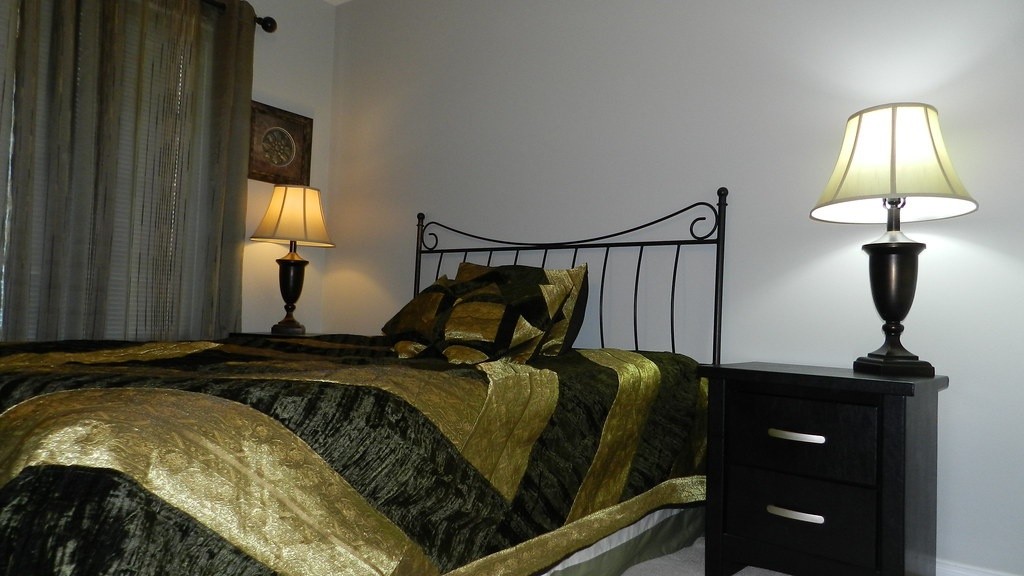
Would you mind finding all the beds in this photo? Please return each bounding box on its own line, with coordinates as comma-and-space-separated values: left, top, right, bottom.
0, 186, 729, 576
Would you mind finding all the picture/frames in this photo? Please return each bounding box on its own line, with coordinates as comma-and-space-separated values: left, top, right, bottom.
248, 99, 313, 186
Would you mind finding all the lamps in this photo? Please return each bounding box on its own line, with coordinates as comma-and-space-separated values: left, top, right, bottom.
810, 103, 980, 379
248, 185, 335, 333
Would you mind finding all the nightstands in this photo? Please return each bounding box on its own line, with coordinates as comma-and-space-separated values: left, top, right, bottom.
699, 362, 949, 576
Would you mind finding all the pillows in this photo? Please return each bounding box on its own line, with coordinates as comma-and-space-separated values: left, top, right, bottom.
382, 274, 462, 358
457, 262, 590, 359
431, 281, 544, 371
502, 283, 575, 365
